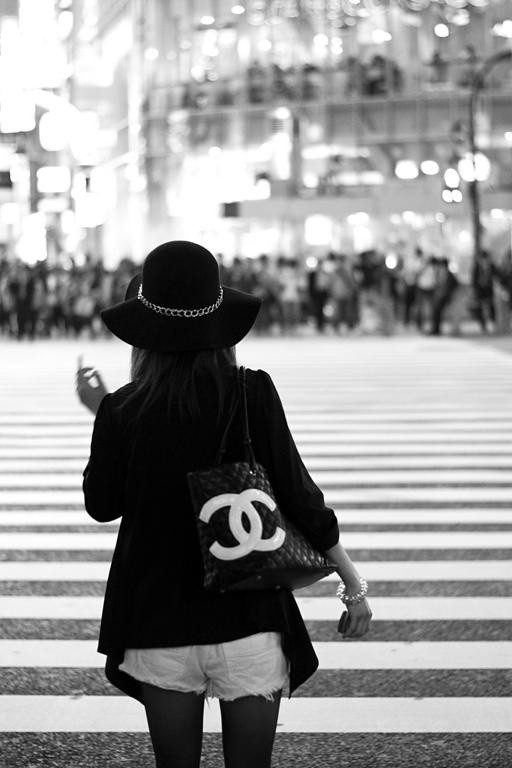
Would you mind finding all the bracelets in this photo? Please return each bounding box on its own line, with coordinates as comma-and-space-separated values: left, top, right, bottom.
335, 578, 368, 604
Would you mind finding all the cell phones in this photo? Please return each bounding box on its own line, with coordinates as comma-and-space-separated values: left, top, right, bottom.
339, 611, 351, 633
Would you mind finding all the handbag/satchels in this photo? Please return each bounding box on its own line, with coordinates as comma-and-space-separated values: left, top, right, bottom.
185, 366, 339, 588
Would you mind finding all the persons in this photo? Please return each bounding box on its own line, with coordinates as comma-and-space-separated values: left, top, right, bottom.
76, 242, 373, 768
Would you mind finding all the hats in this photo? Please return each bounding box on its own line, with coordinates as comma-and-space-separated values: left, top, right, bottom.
100, 241, 263, 353
